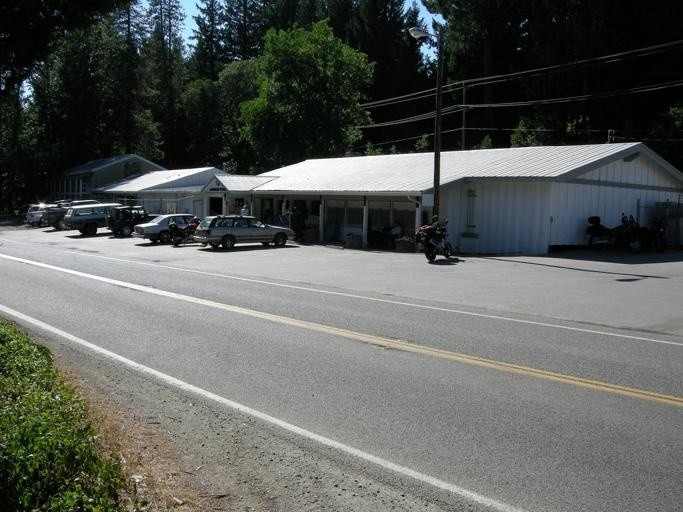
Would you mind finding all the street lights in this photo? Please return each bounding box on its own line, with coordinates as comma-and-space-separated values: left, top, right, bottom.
408, 26, 445, 222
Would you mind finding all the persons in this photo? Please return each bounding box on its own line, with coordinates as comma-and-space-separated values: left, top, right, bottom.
291, 207, 304, 242
240, 201, 250, 215
376, 220, 402, 251
629, 214, 637, 224
622, 213, 628, 225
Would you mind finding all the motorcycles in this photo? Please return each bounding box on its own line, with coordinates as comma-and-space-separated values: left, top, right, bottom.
415, 219, 452, 262
169, 217, 208, 246
587, 213, 667, 255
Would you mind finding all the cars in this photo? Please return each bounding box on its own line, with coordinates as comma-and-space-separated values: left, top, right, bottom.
134, 213, 218, 244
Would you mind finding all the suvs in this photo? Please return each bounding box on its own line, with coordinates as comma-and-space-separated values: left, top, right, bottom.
193, 215, 296, 249
17, 200, 160, 236
